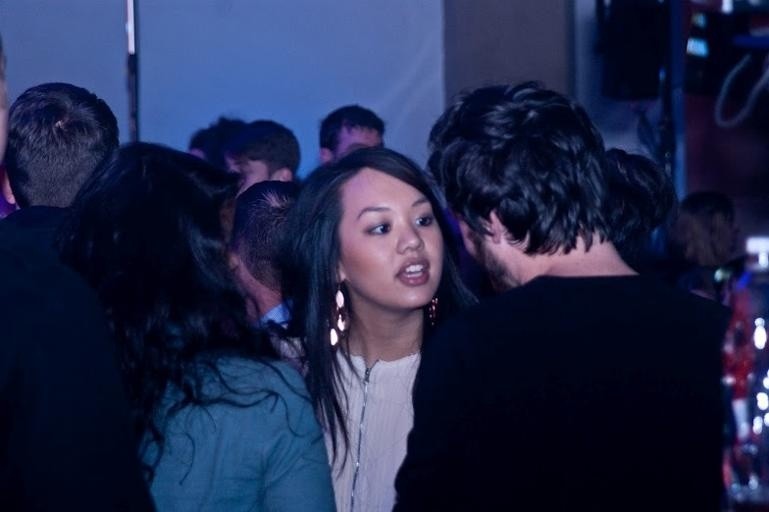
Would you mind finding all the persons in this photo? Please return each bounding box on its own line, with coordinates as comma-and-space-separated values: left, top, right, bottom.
395, 78, 730, 512
62, 141, 339, 510
270, 147, 500, 512
319, 104, 385, 165
229, 180, 309, 381
186, 118, 248, 172
603, 149, 769, 508
1, 83, 154, 511
217, 121, 302, 243
